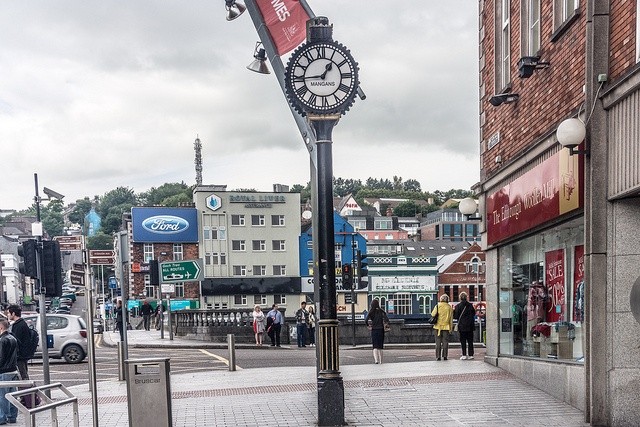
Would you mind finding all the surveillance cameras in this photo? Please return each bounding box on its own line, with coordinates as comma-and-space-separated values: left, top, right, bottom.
40, 187, 64, 201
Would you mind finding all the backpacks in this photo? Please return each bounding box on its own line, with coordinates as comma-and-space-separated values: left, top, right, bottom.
14, 323, 38, 360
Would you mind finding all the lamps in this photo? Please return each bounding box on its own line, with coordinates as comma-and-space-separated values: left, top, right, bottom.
246, 47, 270, 76
222, 0, 247, 22
557, 117, 589, 158
458, 197, 482, 222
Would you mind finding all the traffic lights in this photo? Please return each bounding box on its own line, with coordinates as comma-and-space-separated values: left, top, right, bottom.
357, 250, 368, 289
18, 238, 40, 279
342, 264, 352, 289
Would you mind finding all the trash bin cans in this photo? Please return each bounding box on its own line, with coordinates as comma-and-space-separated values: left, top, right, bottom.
123, 357, 173, 427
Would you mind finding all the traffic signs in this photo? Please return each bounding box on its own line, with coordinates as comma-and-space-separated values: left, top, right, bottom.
160, 260, 202, 283
60, 243, 83, 251
53, 236, 83, 243
90, 257, 116, 265
89, 250, 115, 257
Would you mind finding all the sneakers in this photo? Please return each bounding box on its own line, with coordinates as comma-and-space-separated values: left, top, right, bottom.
444, 357, 447, 359
276, 344, 281, 346
310, 343, 312, 346
313, 344, 316, 346
0, 419, 7, 424
468, 356, 473, 360
271, 344, 275, 345
437, 357, 441, 360
298, 344, 301, 347
7, 418, 15, 423
460, 355, 466, 360
302, 345, 306, 346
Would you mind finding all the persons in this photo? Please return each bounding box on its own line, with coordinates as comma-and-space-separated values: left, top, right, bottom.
265, 304, 284, 347
431, 292, 454, 360
306, 305, 316, 347
140, 299, 154, 330
295, 301, 309, 347
7, 304, 33, 404
365, 299, 389, 363
453, 291, 476, 359
253, 304, 265, 344
0, 316, 18, 425
115, 299, 128, 341
151, 300, 165, 330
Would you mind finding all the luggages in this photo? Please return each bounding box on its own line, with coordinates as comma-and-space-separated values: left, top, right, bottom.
21, 378, 41, 407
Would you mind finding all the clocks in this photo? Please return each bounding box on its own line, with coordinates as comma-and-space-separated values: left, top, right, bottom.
284, 38, 361, 117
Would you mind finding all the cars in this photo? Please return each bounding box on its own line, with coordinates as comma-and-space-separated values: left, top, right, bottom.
50, 282, 76, 313
9, 313, 87, 363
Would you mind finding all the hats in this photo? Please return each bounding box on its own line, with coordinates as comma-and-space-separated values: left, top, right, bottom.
529, 280, 542, 286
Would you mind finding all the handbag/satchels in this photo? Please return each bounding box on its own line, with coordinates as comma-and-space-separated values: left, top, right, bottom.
382, 318, 391, 332
427, 314, 438, 324
454, 321, 460, 331
